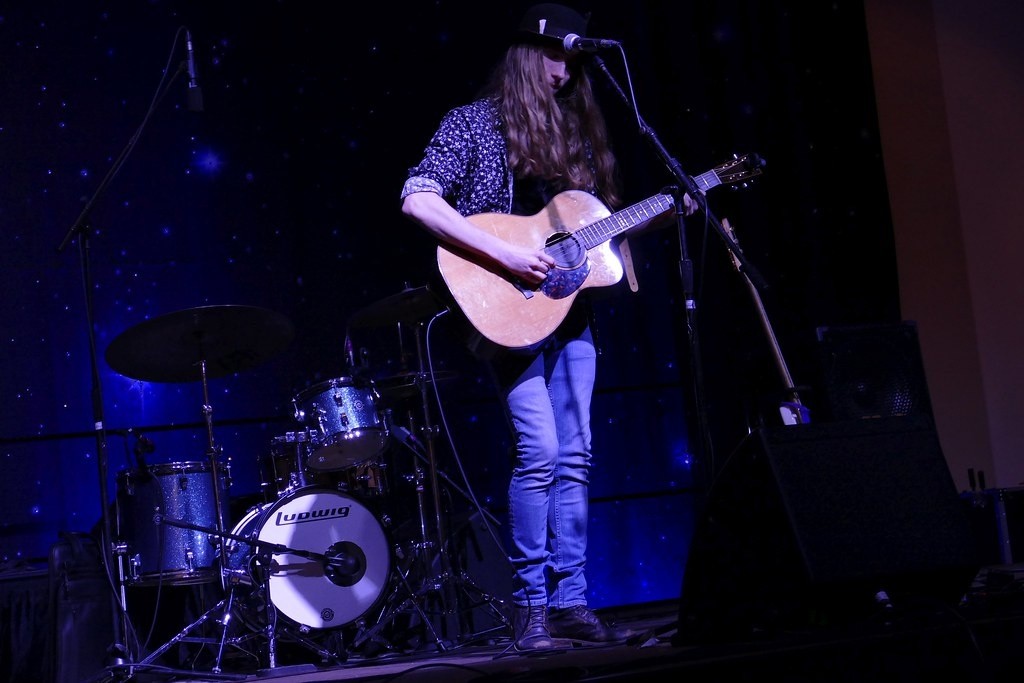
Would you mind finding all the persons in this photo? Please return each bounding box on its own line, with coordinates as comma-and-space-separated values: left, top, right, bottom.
401, 4, 707, 655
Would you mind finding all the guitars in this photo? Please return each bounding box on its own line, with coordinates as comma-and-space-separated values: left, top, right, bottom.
434, 147, 762, 352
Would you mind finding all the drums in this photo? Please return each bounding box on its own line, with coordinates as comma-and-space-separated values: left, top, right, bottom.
119, 454, 232, 588
219, 485, 397, 646
285, 376, 390, 470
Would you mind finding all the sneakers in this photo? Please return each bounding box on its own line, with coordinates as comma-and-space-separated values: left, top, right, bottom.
512, 604, 554, 652
544, 605, 636, 650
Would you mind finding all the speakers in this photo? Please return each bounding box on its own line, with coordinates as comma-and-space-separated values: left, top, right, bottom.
667, 411, 971, 653
814, 320, 938, 429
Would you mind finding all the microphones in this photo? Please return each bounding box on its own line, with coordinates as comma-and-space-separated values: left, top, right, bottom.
185, 33, 199, 90
563, 33, 617, 55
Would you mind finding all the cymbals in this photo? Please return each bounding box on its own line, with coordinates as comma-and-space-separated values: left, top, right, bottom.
103, 306, 278, 383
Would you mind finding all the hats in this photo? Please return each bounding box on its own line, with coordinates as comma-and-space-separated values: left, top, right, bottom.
495, 3, 598, 56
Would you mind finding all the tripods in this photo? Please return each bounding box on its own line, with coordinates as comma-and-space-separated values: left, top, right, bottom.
55, 62, 516, 683
